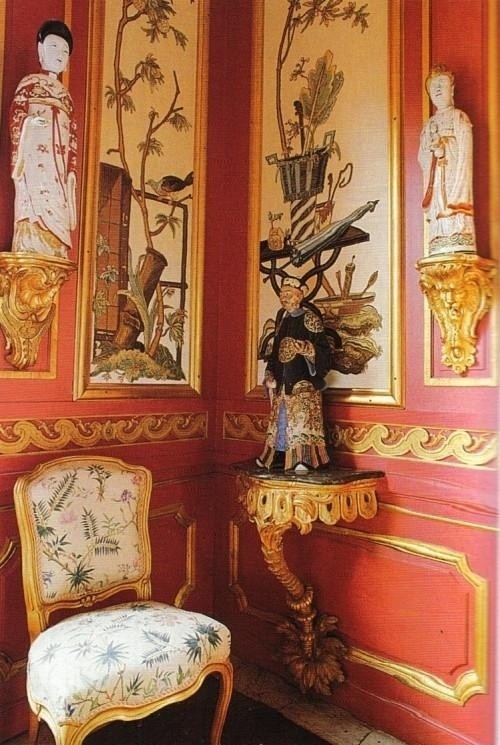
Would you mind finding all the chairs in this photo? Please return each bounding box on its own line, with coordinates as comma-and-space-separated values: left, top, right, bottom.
12, 455, 234, 745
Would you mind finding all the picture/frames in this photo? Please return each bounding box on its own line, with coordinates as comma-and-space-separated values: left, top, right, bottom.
244, 0, 407, 409
73, 0, 210, 401
